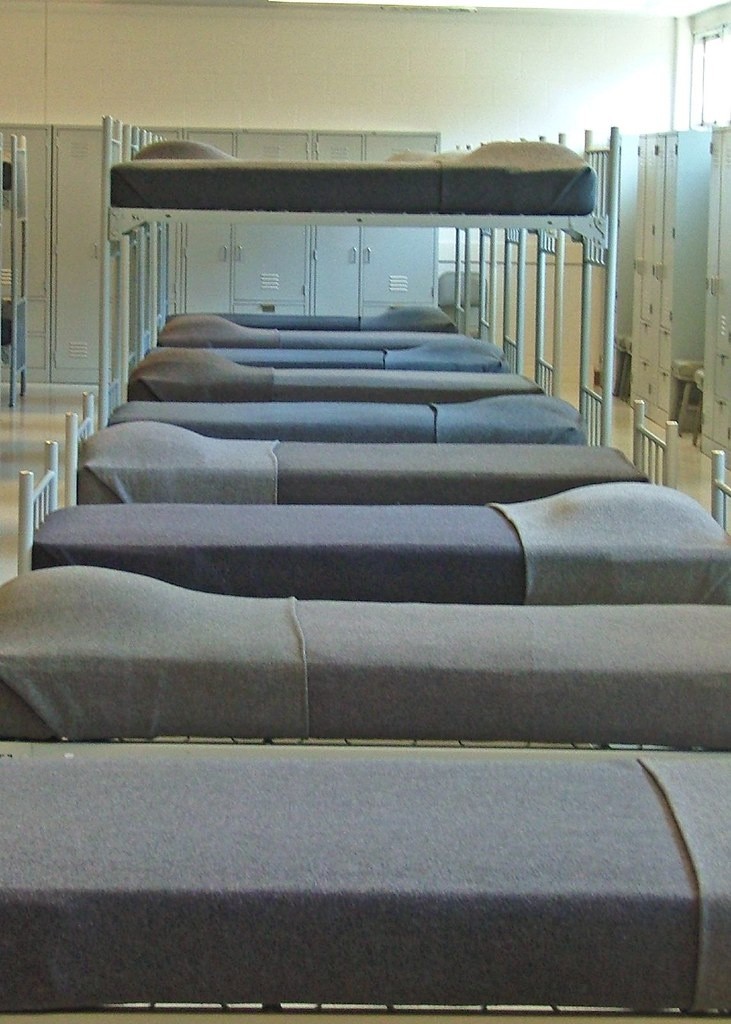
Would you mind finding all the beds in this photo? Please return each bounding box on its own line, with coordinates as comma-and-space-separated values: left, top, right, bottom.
0, 117, 731, 1024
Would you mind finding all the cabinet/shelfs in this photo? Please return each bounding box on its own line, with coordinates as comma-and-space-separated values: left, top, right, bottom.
51, 125, 180, 385
181, 128, 313, 315
699, 126, 731, 471
312, 130, 442, 318
631, 131, 713, 424
0, 121, 50, 382
